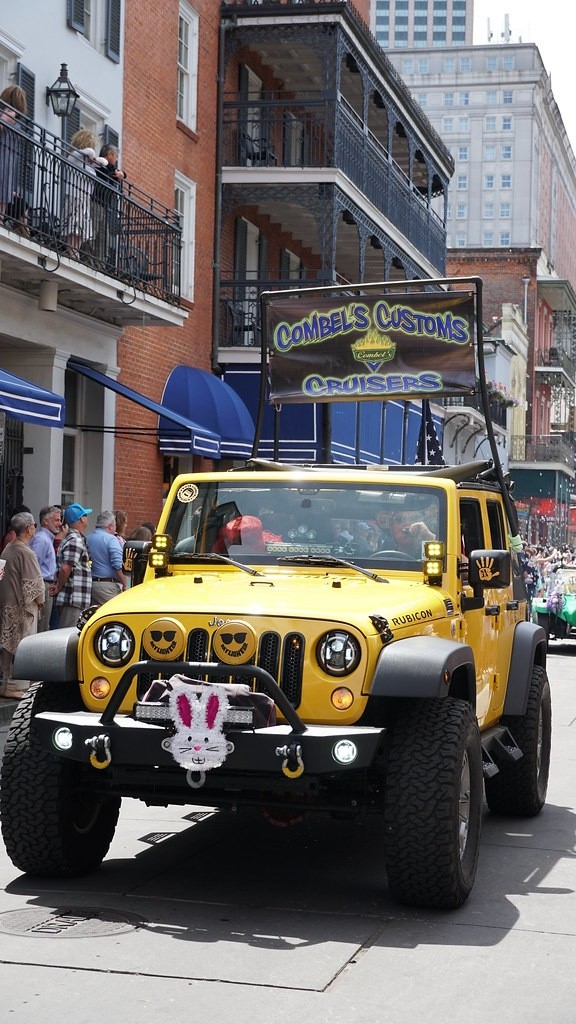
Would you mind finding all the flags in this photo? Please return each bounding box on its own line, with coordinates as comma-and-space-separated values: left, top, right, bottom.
416, 398, 446, 464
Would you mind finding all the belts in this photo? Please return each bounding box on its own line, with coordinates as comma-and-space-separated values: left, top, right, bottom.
43, 579, 54, 584
92, 577, 118, 582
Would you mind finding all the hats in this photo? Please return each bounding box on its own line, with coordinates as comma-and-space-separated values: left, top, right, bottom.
64, 503, 93, 526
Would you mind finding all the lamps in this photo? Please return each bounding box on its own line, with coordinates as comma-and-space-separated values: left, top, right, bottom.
46, 63, 80, 117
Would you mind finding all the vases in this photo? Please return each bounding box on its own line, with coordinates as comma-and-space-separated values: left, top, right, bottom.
476, 373, 519, 407
491, 392, 502, 404
503, 402, 509, 410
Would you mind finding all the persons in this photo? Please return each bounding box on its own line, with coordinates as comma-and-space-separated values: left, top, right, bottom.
86, 143, 126, 270
175, 505, 213, 552
0, 503, 157, 700
522, 542, 576, 598
0, 86, 31, 236
333, 514, 392, 555
61, 129, 108, 260
377, 511, 469, 579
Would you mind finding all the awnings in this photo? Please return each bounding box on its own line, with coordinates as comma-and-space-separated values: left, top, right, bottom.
68, 362, 220, 458
0, 368, 65, 429
157, 366, 254, 460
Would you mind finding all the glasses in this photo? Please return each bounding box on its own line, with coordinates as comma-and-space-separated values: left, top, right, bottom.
25, 522, 37, 530
82, 514, 88, 517
388, 516, 421, 525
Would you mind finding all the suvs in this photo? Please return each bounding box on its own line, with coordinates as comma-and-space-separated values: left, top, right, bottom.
0, 458, 553, 912
527, 563, 576, 651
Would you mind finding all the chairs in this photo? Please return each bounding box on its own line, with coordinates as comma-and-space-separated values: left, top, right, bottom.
120, 244, 166, 290
238, 127, 278, 167
223, 302, 261, 346
